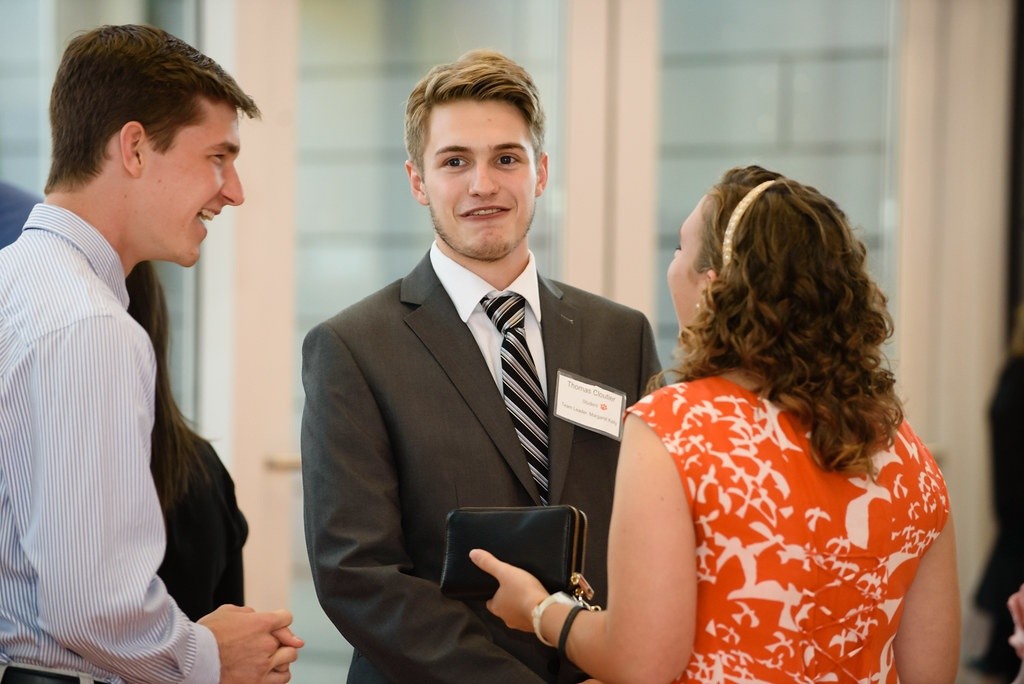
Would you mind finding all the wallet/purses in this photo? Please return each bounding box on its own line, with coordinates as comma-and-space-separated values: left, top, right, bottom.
438, 504, 596, 603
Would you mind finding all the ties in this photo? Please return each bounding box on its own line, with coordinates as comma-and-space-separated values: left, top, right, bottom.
480, 293, 566, 504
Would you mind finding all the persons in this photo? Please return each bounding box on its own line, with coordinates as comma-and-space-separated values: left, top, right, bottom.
1, 25, 306, 684
470, 166, 962, 683
301, 50, 669, 684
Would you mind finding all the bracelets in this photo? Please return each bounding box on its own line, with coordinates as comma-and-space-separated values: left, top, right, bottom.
532, 590, 577, 646
